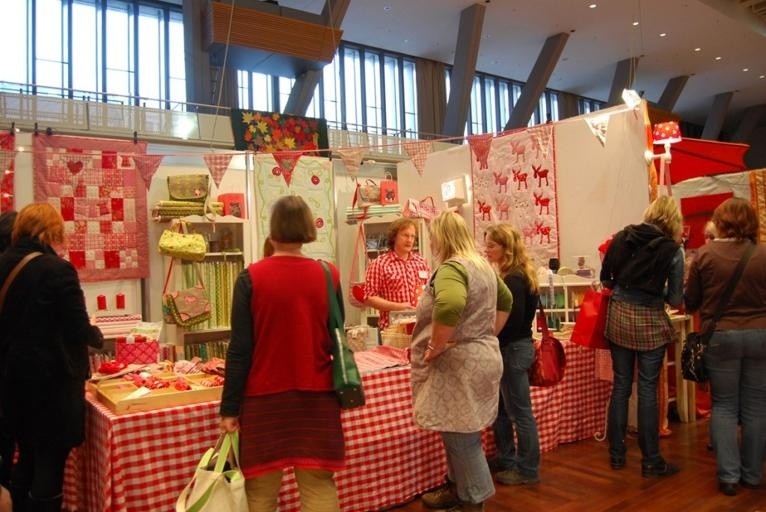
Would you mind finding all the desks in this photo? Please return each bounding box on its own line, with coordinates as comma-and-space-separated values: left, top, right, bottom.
60, 336, 613, 511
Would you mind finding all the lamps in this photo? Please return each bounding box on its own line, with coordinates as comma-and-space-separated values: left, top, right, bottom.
644, 120, 682, 165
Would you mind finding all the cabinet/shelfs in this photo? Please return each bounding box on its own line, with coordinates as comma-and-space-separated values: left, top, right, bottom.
356, 214, 429, 328
662, 316, 697, 425
529, 275, 600, 339
165, 214, 246, 345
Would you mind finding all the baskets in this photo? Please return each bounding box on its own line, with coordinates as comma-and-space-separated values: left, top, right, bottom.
380, 329, 413, 350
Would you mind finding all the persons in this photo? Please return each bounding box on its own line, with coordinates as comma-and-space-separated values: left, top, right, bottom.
1, 208, 17, 254
482, 222, 542, 486
407, 209, 515, 512
217, 194, 346, 512
600, 193, 686, 479
682, 196, 766, 495
0, 200, 106, 512
363, 218, 432, 345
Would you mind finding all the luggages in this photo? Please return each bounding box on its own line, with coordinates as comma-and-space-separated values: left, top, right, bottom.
344, 322, 368, 352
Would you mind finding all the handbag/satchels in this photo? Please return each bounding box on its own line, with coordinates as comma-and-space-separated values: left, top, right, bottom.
162, 282, 213, 329
526, 330, 568, 387
330, 324, 365, 411
678, 329, 711, 383
346, 279, 369, 308
175, 431, 251, 512
355, 183, 382, 209
379, 180, 400, 206
569, 287, 611, 352
167, 173, 211, 204
403, 194, 441, 223
158, 228, 208, 263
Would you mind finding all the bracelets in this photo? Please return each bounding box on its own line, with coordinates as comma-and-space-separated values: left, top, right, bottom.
427, 343, 442, 354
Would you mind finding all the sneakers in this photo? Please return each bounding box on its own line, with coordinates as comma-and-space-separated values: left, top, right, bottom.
485, 455, 517, 471
494, 467, 541, 486
437, 500, 486, 512
608, 455, 628, 469
637, 461, 679, 478
720, 483, 738, 497
421, 482, 459, 508
